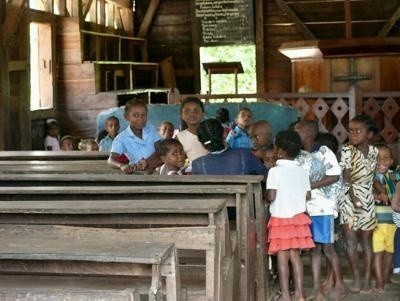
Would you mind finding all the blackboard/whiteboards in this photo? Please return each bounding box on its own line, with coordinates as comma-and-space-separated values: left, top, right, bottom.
194, 0, 255, 46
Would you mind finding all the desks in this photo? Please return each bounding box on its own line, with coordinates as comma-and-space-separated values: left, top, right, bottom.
0, 145, 268, 301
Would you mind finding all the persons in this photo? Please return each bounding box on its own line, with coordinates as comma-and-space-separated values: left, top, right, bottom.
338, 115, 380, 294
315, 132, 339, 153
43, 97, 277, 176
391, 180, 399, 274
294, 119, 346, 300
265, 129, 312, 300
372, 143, 399, 290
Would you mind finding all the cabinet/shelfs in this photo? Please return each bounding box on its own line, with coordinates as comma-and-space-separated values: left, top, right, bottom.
60, 18, 169, 137
289, 51, 399, 129
